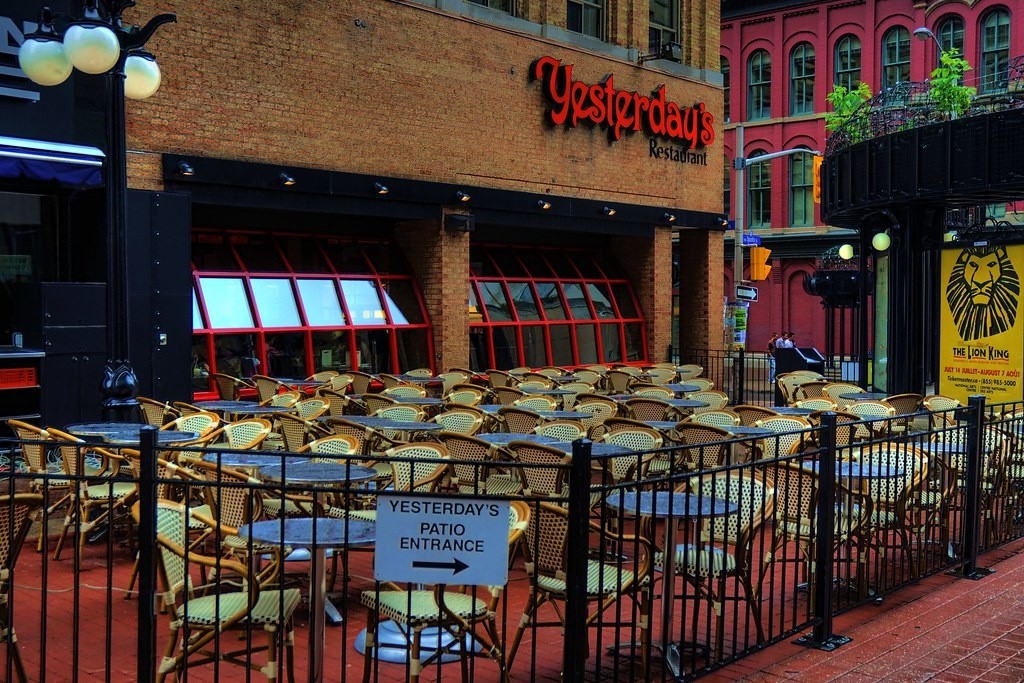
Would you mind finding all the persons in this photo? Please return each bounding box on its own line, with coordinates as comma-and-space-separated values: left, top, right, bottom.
767, 332, 797, 383
253, 342, 274, 377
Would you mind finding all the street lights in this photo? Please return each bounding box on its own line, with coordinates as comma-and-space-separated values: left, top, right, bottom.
838, 227, 893, 391
732, 125, 820, 350
19, 0, 178, 424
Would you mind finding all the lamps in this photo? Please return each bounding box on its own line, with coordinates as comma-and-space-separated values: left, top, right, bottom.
457, 191, 470, 201
872, 228, 891, 251
535, 199, 551, 210
603, 206, 616, 215
373, 181, 388, 194
717, 217, 728, 226
637, 41, 683, 64
275, 169, 295, 185
664, 213, 676, 221
838, 244, 854, 260
912, 28, 956, 72
174, 158, 195, 175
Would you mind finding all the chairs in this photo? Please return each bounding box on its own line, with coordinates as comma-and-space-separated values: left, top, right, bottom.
0, 364, 1024, 683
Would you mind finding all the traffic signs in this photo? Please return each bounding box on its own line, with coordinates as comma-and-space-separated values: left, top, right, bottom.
373, 490, 510, 587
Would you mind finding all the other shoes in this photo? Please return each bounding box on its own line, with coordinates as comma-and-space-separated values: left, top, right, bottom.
768, 380, 775, 383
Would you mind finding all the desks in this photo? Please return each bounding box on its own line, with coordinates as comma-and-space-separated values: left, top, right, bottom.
661, 398, 711, 422
347, 393, 401, 400
105, 431, 200, 444
259, 463, 377, 503
474, 433, 560, 445
795, 460, 903, 606
717, 425, 773, 434
532, 409, 594, 422
363, 420, 445, 441
906, 441, 994, 562
550, 375, 581, 399
395, 375, 445, 388
840, 392, 902, 400
670, 370, 691, 384
315, 415, 394, 455
603, 491, 738, 683
65, 424, 147, 470
523, 389, 578, 397
193, 401, 258, 443
237, 516, 375, 683
279, 380, 325, 391
633, 373, 659, 380
398, 397, 443, 406
221, 406, 293, 419
542, 442, 635, 456
476, 404, 534, 433
659, 384, 701, 398
202, 453, 312, 481
768, 406, 817, 417
643, 420, 682, 461
607, 394, 660, 403
